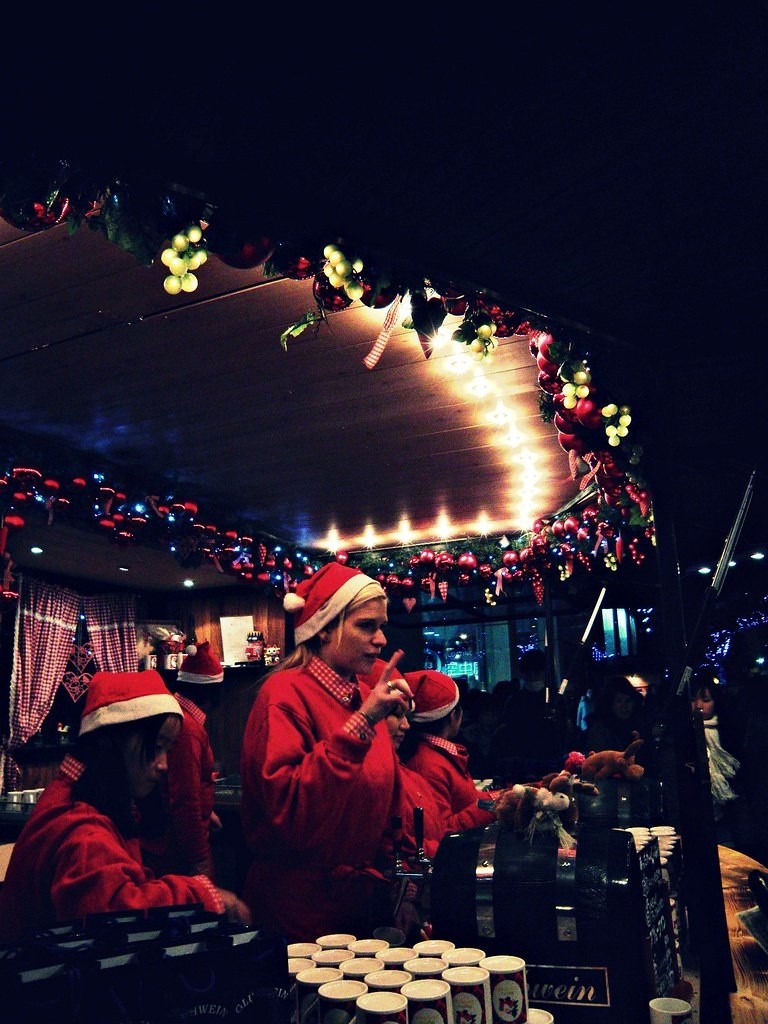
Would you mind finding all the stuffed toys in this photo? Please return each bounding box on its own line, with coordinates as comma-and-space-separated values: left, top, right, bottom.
493, 784, 570, 839
565, 731, 644, 781
541, 770, 599, 797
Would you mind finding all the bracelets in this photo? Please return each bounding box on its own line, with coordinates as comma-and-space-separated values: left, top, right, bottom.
358, 709, 376, 724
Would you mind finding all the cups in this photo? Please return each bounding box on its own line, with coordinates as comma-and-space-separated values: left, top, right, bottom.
649, 998, 692, 1024
5, 788, 46, 812
287, 934, 554, 1024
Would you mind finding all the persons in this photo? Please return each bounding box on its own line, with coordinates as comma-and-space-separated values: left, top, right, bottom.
352, 656, 497, 940
404, 670, 560, 831
0, 667, 251, 948
135, 641, 225, 883
452, 651, 768, 849
239, 562, 415, 942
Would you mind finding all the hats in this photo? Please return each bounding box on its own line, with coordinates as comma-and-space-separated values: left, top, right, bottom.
358, 658, 410, 696
176, 641, 225, 683
283, 562, 380, 647
78, 670, 185, 738
403, 670, 459, 722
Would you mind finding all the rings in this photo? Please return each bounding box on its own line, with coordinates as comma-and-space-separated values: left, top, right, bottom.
386, 680, 392, 688
